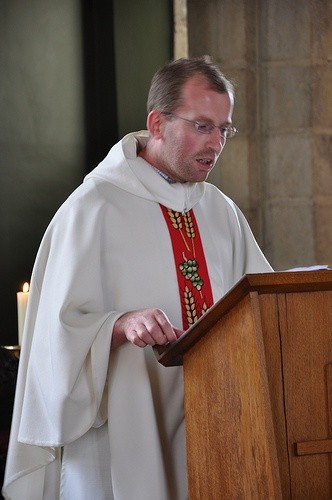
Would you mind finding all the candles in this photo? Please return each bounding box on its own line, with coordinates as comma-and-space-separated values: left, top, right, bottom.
17, 282, 29, 346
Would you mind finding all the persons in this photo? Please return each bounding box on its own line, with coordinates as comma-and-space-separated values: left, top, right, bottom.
2, 56, 274, 499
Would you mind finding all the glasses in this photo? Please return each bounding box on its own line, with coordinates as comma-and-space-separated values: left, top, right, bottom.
162, 111, 238, 139
